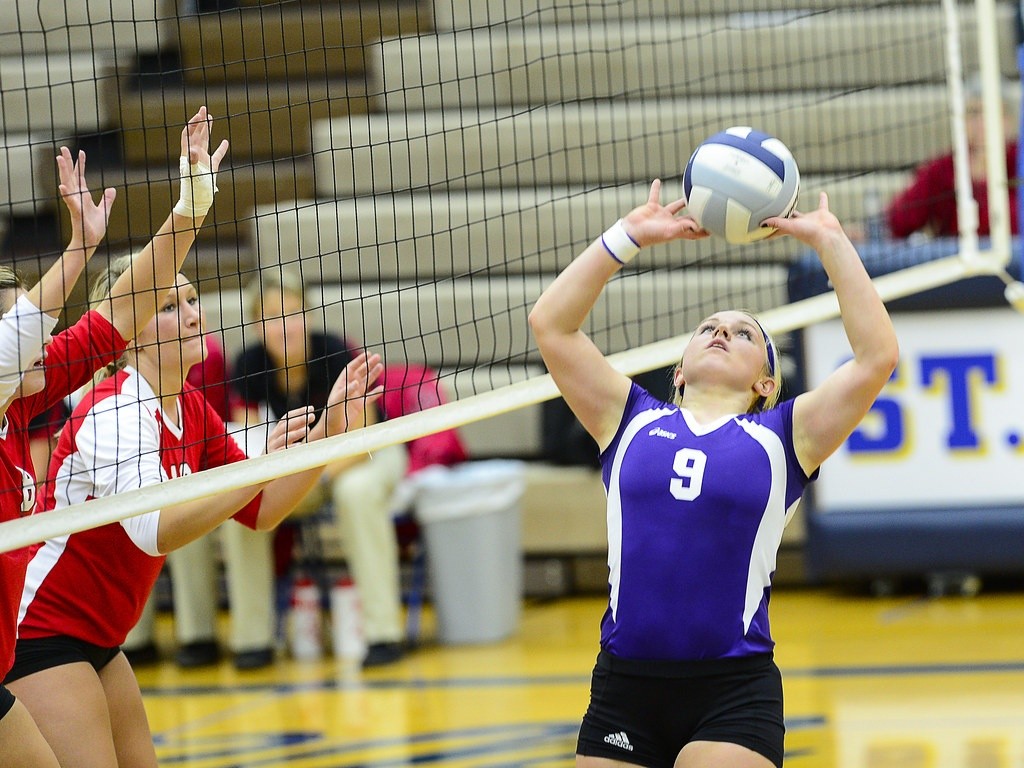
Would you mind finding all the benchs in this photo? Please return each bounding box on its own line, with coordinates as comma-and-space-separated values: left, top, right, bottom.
2, 0, 1024, 458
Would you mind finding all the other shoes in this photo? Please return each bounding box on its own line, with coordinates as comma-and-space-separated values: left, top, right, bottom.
361, 640, 404, 667
175, 635, 223, 670
232, 648, 274, 671
125, 639, 167, 668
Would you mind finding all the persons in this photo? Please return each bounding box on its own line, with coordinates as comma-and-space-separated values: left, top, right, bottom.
1, 98, 472, 768
522, 174, 901, 766
884, 59, 1022, 242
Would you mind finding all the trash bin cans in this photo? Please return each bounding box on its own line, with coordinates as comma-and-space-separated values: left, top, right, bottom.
413, 457, 527, 645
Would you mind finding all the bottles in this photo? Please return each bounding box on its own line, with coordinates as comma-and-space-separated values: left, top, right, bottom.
294, 579, 321, 659
328, 579, 366, 660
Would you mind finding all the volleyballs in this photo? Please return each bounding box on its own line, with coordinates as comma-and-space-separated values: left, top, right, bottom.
679, 124, 804, 246
